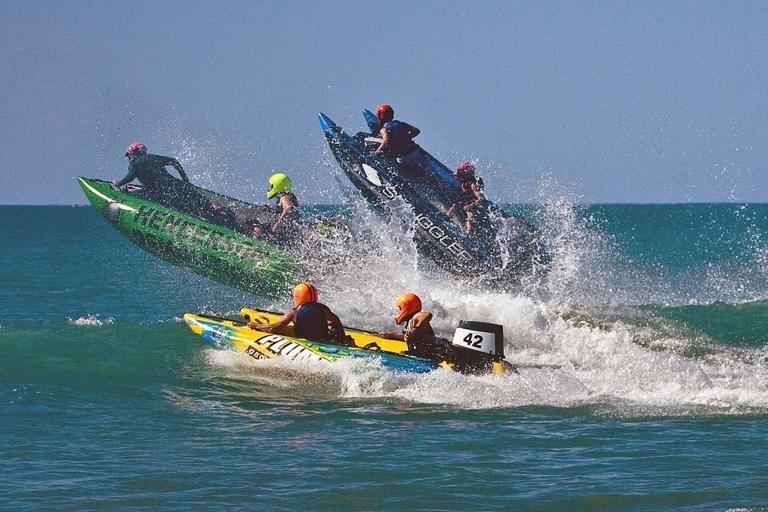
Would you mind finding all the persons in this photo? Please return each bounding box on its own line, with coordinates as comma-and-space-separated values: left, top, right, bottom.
112, 142, 190, 203
247, 280, 330, 342
370, 104, 428, 177
377, 293, 437, 358
252, 173, 304, 250
445, 162, 484, 237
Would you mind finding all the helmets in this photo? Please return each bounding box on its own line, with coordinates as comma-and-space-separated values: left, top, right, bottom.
266, 174, 292, 199
394, 292, 421, 325
375, 104, 394, 123
123, 142, 147, 162
456, 161, 475, 182
293, 283, 318, 308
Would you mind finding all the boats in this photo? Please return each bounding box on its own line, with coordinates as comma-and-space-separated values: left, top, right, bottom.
320, 108, 549, 290
184, 305, 518, 383
77, 174, 348, 299
